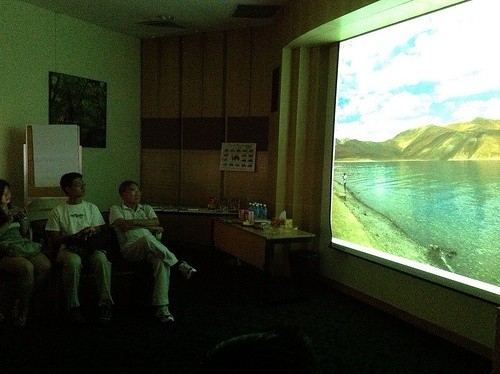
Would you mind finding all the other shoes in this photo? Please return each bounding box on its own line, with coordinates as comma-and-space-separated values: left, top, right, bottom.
99, 306, 112, 321
70, 309, 85, 323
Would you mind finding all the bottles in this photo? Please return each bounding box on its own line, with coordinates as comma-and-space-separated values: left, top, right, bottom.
236, 193, 267, 223
208, 195, 216, 213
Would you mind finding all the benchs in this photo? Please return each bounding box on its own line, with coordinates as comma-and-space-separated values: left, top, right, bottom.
39, 223, 140, 296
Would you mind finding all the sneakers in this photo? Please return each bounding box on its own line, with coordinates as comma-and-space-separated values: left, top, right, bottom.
179, 260, 197, 279
154, 306, 174, 321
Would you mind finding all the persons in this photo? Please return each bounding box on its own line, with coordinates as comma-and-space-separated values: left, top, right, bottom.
108, 180, 197, 324
200, 328, 317, 374
0, 178, 52, 327
45, 173, 114, 321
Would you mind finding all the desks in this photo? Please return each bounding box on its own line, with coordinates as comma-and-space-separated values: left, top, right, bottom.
151, 205, 239, 245
213, 218, 316, 282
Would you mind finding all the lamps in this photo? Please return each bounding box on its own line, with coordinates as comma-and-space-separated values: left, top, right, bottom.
138, 14, 187, 29
